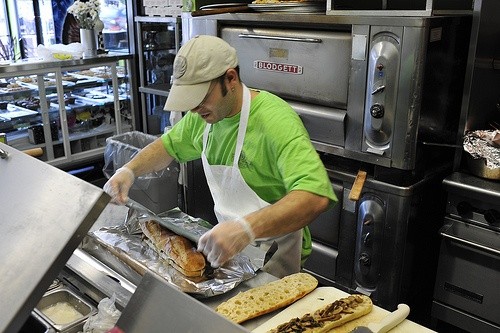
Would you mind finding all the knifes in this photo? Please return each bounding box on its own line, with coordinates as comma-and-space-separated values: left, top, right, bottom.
350, 302, 411, 332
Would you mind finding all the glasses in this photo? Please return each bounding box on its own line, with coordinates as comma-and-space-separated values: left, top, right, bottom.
193, 79, 219, 111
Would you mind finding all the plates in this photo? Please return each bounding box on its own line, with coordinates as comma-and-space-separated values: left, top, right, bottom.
199, 1, 319, 13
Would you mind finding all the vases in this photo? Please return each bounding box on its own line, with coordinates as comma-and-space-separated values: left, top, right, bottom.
79, 28, 98, 57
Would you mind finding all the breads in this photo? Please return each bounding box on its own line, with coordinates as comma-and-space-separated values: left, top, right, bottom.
252, 0, 280, 4
139, 220, 205, 277
270, 294, 373, 333
20, 148, 43, 156
6, 70, 109, 89
216, 272, 318, 323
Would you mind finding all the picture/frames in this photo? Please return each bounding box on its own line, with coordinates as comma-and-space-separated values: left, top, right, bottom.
67, 0, 105, 32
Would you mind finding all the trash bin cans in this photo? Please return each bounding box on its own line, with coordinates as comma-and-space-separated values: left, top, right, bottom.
106, 129, 179, 214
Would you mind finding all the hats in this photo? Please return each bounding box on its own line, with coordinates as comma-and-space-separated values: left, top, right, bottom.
163, 34, 238, 111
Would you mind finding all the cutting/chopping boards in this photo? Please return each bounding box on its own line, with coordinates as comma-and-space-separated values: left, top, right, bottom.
251, 285, 438, 333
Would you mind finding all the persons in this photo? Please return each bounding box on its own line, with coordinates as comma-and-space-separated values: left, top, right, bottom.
102, 34, 338, 280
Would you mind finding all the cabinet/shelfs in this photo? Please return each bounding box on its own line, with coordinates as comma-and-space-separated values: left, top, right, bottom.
8, 122, 131, 160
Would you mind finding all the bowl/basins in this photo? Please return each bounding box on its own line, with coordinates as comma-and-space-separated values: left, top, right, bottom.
465, 128, 500, 180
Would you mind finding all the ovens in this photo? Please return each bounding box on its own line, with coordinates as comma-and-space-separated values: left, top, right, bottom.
179, 8, 473, 310
